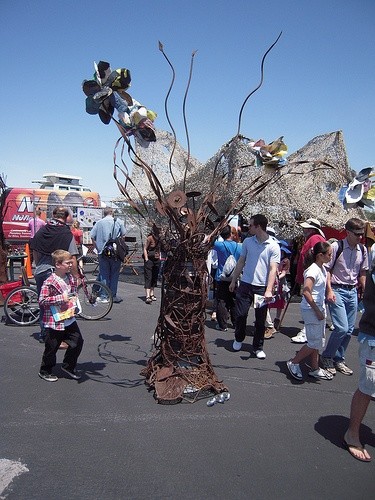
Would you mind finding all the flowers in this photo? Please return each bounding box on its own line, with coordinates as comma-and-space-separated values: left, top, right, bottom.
82, 30, 375, 251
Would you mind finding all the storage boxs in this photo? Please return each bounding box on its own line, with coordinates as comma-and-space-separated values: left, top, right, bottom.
0, 280, 23, 305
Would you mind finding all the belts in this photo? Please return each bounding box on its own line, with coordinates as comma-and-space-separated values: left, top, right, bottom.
331, 283, 356, 290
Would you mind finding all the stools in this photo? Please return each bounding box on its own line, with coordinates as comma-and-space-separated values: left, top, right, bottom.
6, 254, 28, 282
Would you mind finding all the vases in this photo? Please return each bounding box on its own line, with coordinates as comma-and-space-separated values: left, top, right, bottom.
139, 251, 231, 405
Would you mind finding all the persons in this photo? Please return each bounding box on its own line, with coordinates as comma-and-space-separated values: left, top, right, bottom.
209, 218, 375, 349
71, 221, 84, 277
343, 266, 375, 462
322, 218, 369, 377
30, 206, 79, 348
285, 241, 334, 381
91, 207, 126, 304
229, 214, 280, 360
143, 226, 160, 304
27, 210, 48, 263
38, 250, 85, 382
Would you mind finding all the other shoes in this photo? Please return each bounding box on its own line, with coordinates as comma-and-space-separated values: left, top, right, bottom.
211, 313, 217, 321
215, 324, 228, 331
38, 369, 58, 382
90, 293, 125, 304
61, 364, 81, 379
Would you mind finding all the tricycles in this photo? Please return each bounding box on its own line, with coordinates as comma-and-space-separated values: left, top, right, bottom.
0, 255, 114, 326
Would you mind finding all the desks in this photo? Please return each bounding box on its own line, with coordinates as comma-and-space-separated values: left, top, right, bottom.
119, 248, 138, 276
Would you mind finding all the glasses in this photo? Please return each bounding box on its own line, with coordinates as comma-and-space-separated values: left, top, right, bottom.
346, 228, 365, 237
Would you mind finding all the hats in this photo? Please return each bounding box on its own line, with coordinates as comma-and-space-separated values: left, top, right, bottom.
266, 227, 281, 244
300, 218, 326, 239
279, 239, 292, 256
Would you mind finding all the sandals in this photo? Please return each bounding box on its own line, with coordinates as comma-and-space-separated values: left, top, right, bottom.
145, 294, 157, 304
342, 438, 372, 462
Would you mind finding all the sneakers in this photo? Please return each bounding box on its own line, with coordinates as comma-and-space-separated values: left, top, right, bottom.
286, 359, 303, 381
333, 358, 354, 376
252, 349, 266, 359
290, 325, 308, 343
308, 366, 333, 380
319, 355, 337, 376
263, 327, 276, 339
232, 339, 242, 351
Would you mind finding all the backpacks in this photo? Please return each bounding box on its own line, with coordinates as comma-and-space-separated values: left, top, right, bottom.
218, 241, 240, 282
102, 238, 121, 262
